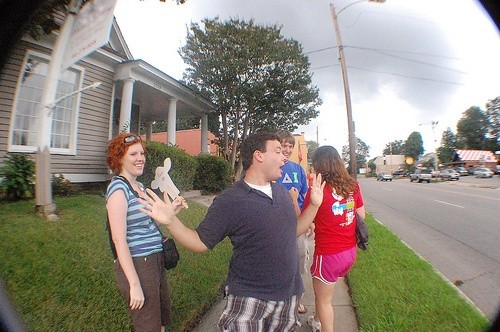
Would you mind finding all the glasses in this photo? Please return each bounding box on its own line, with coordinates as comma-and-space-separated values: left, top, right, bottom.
125, 135, 140, 142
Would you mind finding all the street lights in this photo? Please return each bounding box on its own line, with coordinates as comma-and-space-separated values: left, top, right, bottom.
326, 0, 386, 181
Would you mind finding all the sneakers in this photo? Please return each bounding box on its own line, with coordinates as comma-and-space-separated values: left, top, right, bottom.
306, 315, 321, 332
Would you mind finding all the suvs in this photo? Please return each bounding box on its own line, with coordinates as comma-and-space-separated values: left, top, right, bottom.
409, 167, 432, 183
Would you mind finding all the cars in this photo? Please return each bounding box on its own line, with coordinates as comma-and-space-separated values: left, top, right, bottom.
393, 173, 406, 177
452, 167, 469, 177
491, 164, 500, 174
439, 169, 461, 181
473, 167, 494, 178
468, 167, 476, 174
376, 172, 393, 182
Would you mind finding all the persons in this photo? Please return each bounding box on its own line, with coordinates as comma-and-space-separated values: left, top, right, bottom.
272, 132, 315, 313
105, 133, 187, 332
288, 146, 365, 332
136, 133, 326, 332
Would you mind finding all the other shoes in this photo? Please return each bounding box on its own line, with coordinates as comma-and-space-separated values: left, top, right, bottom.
298, 304, 308, 313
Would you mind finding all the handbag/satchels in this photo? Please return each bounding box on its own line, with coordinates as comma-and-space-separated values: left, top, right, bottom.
355, 213, 369, 251
161, 236, 179, 270
